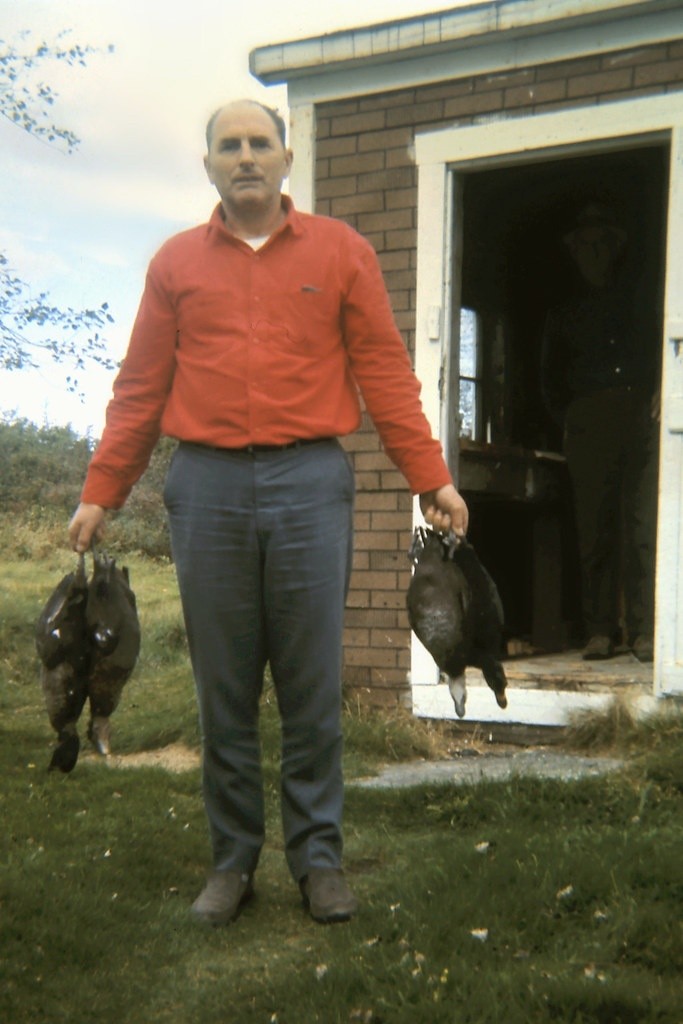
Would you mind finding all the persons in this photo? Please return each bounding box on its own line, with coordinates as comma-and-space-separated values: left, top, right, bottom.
541, 201, 662, 663
67, 100, 470, 927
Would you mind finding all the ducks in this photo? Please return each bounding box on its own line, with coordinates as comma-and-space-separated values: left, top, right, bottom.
33, 548, 101, 774
452, 529, 510, 708
84, 533, 141, 757
405, 526, 479, 719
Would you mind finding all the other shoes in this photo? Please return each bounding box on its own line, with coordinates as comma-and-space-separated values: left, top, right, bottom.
298, 867, 357, 922
585, 631, 655, 661
189, 875, 252, 924
